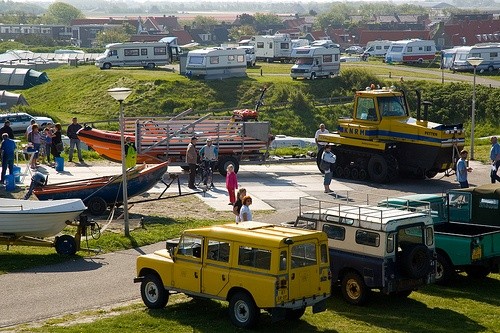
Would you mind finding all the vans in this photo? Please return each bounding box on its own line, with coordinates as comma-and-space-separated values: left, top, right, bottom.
94, 40, 173, 70
151, 28, 340, 80
360, 38, 500, 76
290, 45, 340, 81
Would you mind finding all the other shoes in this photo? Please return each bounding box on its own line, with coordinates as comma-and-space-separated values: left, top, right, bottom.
192, 187, 197, 189
79, 159, 84, 161
325, 190, 333, 193
68, 159, 72, 162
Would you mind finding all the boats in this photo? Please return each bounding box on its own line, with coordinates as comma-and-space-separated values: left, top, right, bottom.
75, 122, 275, 166
23, 159, 172, 208
0, 197, 89, 237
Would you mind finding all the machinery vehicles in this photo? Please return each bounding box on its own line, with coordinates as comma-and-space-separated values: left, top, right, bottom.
316, 83, 465, 184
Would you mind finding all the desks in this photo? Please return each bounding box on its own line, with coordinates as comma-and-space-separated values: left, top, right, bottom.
15, 150, 38, 183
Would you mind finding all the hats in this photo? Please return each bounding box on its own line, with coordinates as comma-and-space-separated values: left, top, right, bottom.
207, 137, 212, 142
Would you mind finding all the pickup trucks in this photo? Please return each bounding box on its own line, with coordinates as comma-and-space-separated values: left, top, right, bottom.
376, 194, 500, 286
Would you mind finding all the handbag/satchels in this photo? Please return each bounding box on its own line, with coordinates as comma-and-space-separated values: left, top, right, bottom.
320, 154, 330, 171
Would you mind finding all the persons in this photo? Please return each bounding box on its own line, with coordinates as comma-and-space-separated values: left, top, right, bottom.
0, 120, 14, 140
315, 123, 329, 151
319, 144, 336, 193
233, 192, 246, 224
199, 137, 218, 188
489, 137, 500, 183
239, 188, 246, 196
239, 195, 252, 222
456, 150, 473, 188
185, 136, 197, 188
225, 164, 238, 205
26, 120, 62, 168
67, 117, 84, 162
0, 133, 16, 184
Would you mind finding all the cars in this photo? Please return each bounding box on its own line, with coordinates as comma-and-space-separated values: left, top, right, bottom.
345, 45, 365, 55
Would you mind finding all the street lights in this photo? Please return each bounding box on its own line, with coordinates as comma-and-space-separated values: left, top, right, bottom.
105, 86, 133, 238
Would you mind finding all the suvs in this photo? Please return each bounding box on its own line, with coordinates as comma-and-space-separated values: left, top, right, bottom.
440, 183, 500, 227
0, 112, 55, 134
281, 196, 440, 306
133, 220, 334, 329
